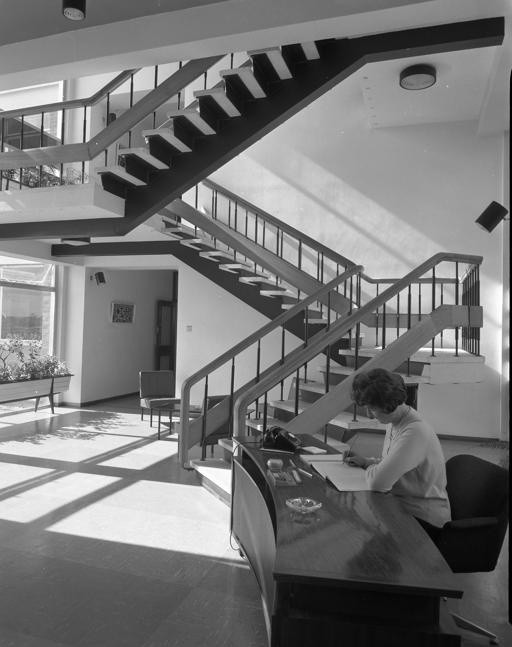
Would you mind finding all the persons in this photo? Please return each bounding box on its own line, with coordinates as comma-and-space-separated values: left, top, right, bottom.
344, 368, 454, 535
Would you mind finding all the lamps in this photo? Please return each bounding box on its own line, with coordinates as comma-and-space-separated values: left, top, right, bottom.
399, 64, 437, 90
63, 0, 86, 21
475, 201, 510, 239
90, 272, 106, 288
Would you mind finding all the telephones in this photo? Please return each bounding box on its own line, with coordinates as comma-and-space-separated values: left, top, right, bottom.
262, 425, 303, 452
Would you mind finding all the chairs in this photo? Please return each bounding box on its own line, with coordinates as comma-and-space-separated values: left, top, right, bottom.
442, 454, 511, 645
138, 369, 181, 440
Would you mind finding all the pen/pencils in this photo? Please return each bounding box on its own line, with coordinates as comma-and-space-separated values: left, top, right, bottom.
297, 468, 313, 477
289, 459, 297, 468
342, 435, 360, 465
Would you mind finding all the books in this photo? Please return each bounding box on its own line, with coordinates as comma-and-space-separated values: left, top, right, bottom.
311, 461, 371, 492
301, 444, 327, 454
298, 453, 343, 466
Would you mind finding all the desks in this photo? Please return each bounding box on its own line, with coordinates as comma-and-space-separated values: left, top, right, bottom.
228, 434, 464, 647
